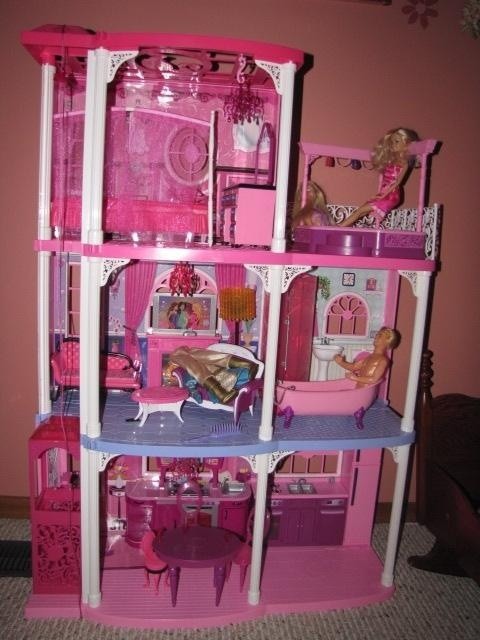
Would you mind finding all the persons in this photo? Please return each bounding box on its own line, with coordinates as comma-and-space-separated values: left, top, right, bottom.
334, 126, 423, 229
16, 24, 444, 633
333, 327, 401, 388
289, 180, 337, 235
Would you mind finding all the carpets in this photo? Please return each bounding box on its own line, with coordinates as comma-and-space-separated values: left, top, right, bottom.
0, 516, 480, 639
